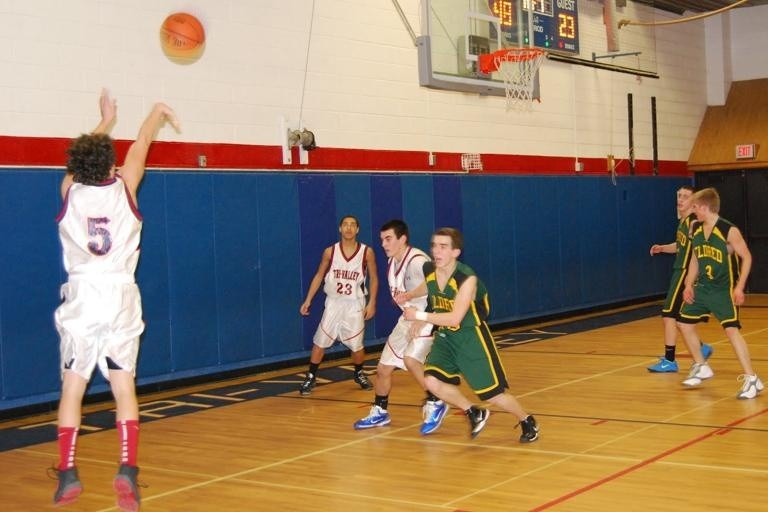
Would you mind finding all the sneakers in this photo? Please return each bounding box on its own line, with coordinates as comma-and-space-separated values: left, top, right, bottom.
647, 355, 678, 372
48, 464, 81, 503
353, 370, 374, 390
736, 374, 764, 398
468, 408, 490, 438
114, 464, 148, 511
514, 415, 540, 442
701, 343, 713, 361
299, 372, 315, 394
420, 400, 449, 433
681, 363, 714, 385
354, 405, 391, 430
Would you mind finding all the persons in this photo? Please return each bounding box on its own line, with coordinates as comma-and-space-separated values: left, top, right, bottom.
49, 86, 185, 510
298, 215, 379, 396
354, 220, 451, 436
680, 187, 765, 399
391, 227, 541, 442
646, 184, 713, 373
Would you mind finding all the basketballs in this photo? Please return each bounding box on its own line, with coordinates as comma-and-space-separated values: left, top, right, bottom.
160, 13, 204, 64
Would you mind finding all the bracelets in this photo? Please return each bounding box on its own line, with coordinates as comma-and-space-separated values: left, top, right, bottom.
415, 311, 428, 321
